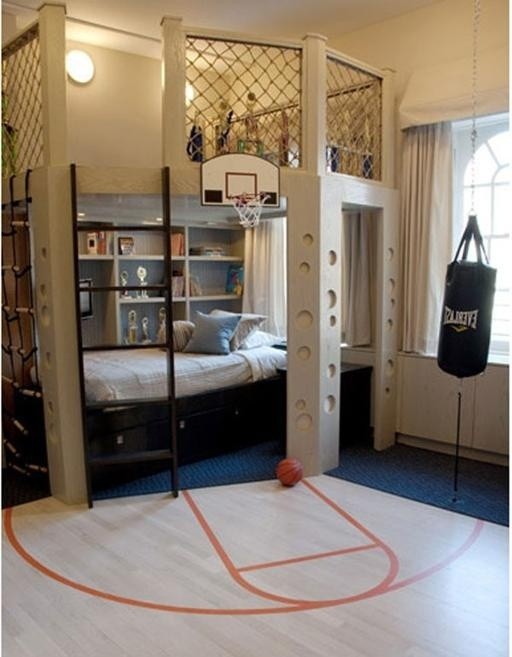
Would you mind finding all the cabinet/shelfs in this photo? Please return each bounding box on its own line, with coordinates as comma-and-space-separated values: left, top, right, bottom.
75, 191, 283, 350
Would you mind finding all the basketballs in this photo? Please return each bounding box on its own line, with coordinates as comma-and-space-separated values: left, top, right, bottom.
276, 458, 303, 485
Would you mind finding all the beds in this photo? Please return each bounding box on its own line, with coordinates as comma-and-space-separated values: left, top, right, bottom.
83, 339, 288, 494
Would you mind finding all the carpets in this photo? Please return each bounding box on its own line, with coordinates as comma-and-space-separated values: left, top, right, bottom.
91, 440, 287, 502
2, 467, 51, 510
323, 437, 509, 526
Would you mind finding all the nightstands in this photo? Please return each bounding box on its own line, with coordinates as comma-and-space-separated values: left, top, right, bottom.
275, 360, 373, 450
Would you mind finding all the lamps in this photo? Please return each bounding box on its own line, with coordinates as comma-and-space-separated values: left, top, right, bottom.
65, 48, 96, 85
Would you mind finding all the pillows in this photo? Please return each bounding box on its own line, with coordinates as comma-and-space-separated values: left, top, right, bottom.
182, 308, 286, 355
